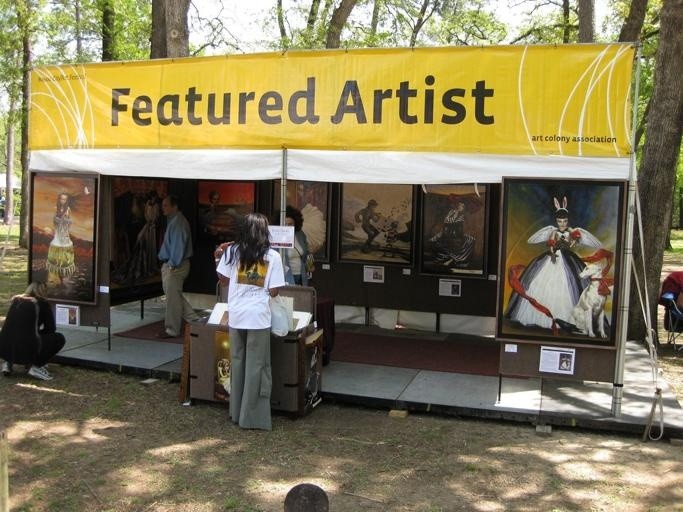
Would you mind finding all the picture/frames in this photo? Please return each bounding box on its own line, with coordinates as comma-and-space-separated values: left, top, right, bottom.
415, 185, 492, 281
496, 176, 629, 351
270, 180, 333, 263
191, 180, 261, 278
28, 170, 102, 306
337, 183, 418, 269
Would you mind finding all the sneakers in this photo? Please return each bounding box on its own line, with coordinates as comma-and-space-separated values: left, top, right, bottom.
155, 329, 176, 338
0, 361, 11, 373
27, 365, 53, 380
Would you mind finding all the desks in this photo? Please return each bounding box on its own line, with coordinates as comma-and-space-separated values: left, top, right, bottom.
177, 310, 324, 420
314, 294, 335, 365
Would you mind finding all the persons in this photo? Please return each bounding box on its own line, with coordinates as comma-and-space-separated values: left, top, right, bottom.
503, 209, 591, 332
274, 204, 318, 285
427, 200, 478, 267
381, 220, 400, 258
355, 199, 380, 253
200, 189, 224, 229
69, 310, 76, 324
215, 212, 288, 432
134, 190, 162, 281
281, 482, 330, 511
45, 191, 82, 283
0, 279, 66, 382
157, 194, 199, 341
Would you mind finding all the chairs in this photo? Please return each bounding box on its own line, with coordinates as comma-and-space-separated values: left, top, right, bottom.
661, 292, 683, 353
